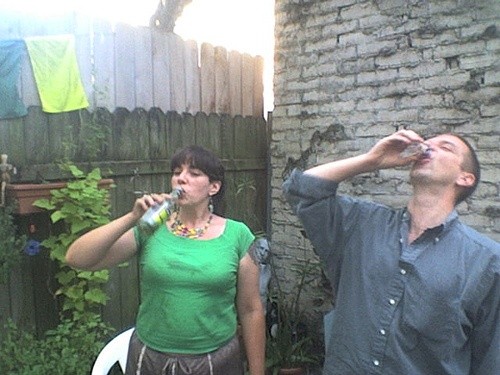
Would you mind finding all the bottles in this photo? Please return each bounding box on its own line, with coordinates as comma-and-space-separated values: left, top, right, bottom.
267, 295, 279, 339
137, 187, 183, 235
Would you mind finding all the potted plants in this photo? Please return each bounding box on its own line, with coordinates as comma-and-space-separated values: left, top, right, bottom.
263, 222, 330, 375
5, 88, 113, 215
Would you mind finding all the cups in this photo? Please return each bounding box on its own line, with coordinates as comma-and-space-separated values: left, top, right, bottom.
407, 140, 431, 160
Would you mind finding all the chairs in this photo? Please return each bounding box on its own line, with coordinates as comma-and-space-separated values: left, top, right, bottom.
91, 327, 135, 375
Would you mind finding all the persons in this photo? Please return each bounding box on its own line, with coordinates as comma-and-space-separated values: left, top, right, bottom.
283, 130, 500, 375
254, 238, 270, 312
172, 212, 212, 238
66, 146, 265, 375
